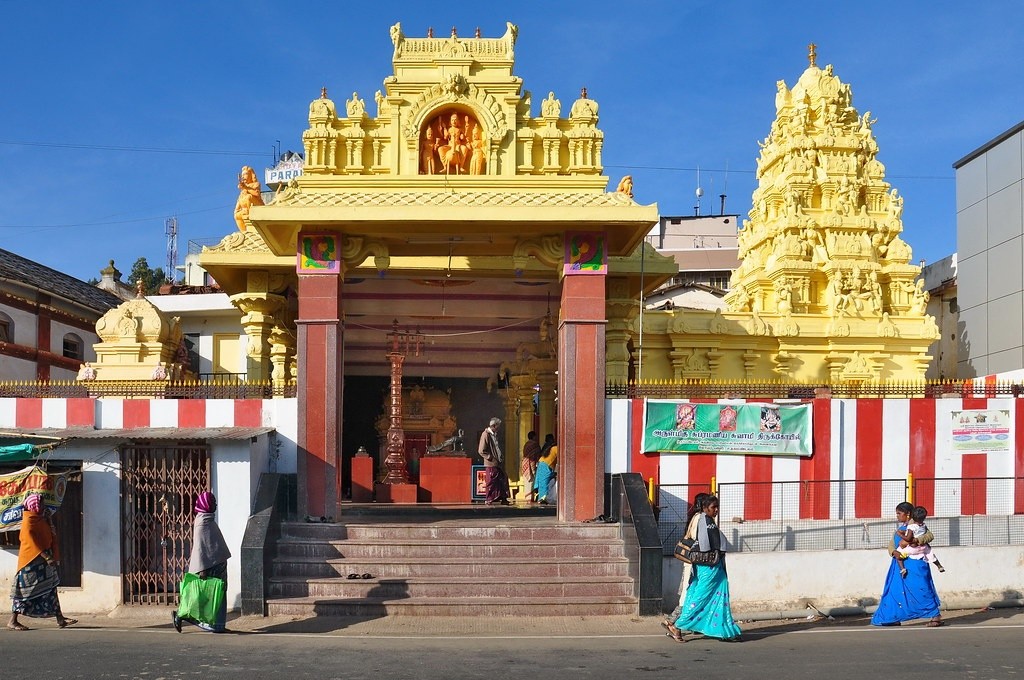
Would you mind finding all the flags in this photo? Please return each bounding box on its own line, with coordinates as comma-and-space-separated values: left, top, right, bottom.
640, 399, 813, 460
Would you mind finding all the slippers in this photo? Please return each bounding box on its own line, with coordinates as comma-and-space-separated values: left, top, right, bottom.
929, 621, 945, 627
212, 628, 231, 632
661, 622, 670, 631
171, 610, 182, 634
8, 625, 29, 631
883, 622, 901, 626
59, 618, 78, 629
664, 626, 685, 643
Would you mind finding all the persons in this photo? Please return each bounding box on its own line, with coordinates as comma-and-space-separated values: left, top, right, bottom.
896, 506, 945, 579
803, 137, 830, 183
737, 219, 753, 248
729, 283, 750, 312
172, 492, 232, 633
661, 493, 744, 642
522, 431, 557, 504
234, 166, 282, 232
827, 264, 883, 314
798, 216, 833, 264
7, 494, 78, 631
782, 173, 904, 221
913, 278, 930, 315
420, 113, 482, 175
772, 275, 792, 313
478, 417, 514, 505
757, 105, 878, 148
872, 502, 945, 626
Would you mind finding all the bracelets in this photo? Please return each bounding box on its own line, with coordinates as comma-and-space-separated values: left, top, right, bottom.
45, 555, 51, 561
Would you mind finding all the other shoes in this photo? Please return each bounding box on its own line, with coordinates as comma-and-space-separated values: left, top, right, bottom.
939, 566, 945, 572
485, 502, 493, 506
501, 501, 510, 505
538, 500, 548, 505
900, 569, 906, 578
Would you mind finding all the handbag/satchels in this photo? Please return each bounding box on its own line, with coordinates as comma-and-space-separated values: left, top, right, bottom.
674, 540, 695, 564
688, 546, 720, 565
